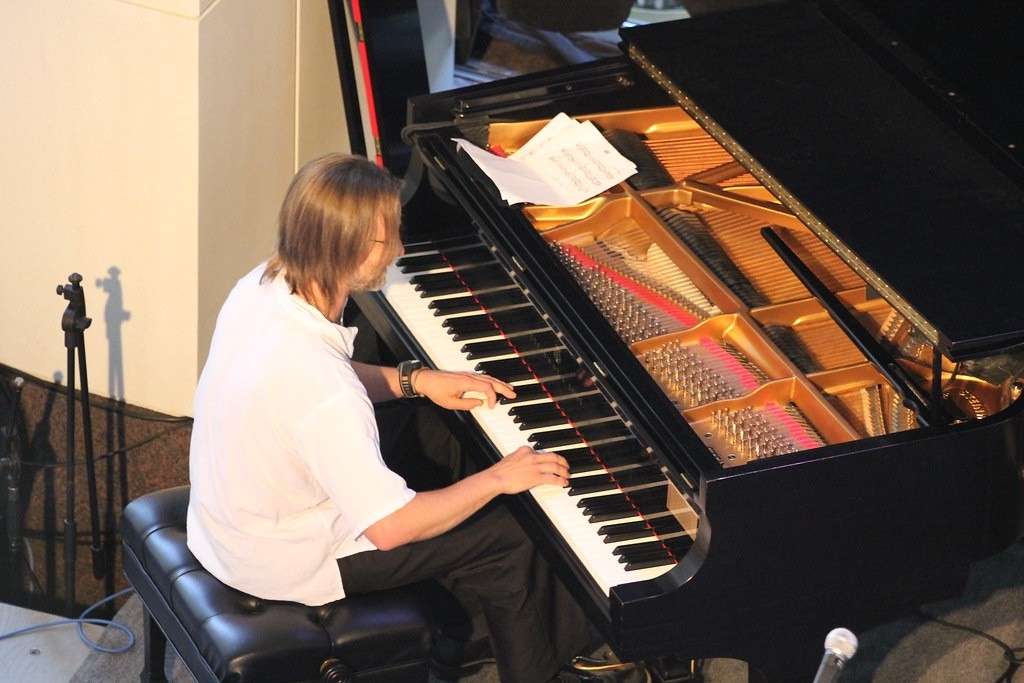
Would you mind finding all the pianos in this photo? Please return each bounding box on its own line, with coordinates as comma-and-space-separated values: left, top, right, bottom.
339, 0, 1024, 683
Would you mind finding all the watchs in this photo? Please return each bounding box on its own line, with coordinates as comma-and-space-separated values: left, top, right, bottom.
397, 359, 424, 398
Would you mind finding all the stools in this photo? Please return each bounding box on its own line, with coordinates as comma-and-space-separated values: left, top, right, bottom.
120, 486, 447, 683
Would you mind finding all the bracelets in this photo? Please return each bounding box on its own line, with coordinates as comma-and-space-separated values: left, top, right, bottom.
412, 367, 429, 397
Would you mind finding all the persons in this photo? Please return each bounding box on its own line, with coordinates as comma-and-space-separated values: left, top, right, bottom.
187, 152, 651, 683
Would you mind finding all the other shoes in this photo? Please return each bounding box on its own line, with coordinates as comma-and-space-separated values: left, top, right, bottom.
545, 665, 652, 683
431, 635, 495, 672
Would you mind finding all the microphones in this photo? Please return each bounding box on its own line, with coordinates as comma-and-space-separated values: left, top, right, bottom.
814, 627, 859, 683
